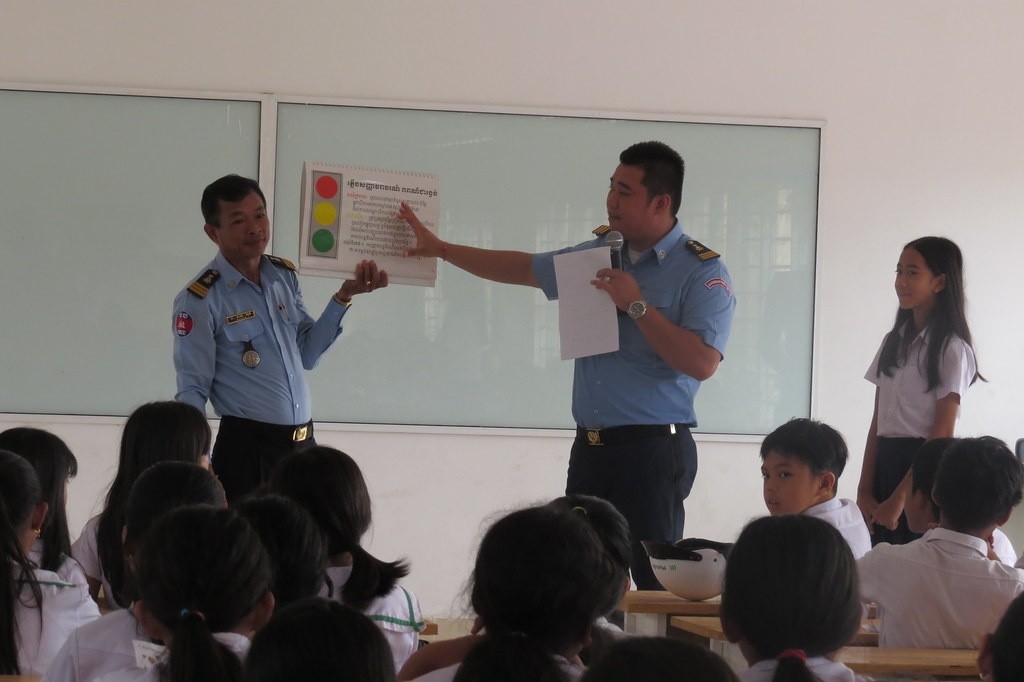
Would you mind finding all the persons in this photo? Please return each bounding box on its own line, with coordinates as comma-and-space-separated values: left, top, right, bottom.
397, 139, 738, 592
0, 400, 1024, 682
857, 235, 989, 547
171, 174, 390, 504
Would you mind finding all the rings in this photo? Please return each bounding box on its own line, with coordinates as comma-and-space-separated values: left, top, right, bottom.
366, 280, 370, 286
603, 275, 611, 282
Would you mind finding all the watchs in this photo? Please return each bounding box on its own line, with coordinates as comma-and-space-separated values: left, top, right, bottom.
627, 299, 648, 319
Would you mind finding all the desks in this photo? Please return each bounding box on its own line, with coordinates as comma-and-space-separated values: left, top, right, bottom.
410, 590, 985, 682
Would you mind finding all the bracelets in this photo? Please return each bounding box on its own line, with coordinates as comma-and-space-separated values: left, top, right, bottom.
338, 288, 352, 302
442, 242, 449, 263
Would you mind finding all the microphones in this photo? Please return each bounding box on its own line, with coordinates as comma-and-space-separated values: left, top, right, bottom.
605, 230, 624, 272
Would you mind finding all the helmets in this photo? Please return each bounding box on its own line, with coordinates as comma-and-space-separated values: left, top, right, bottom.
641, 537, 736, 601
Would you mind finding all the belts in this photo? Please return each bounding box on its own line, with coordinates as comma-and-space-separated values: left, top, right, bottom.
576, 423, 688, 446
221, 416, 312, 443
